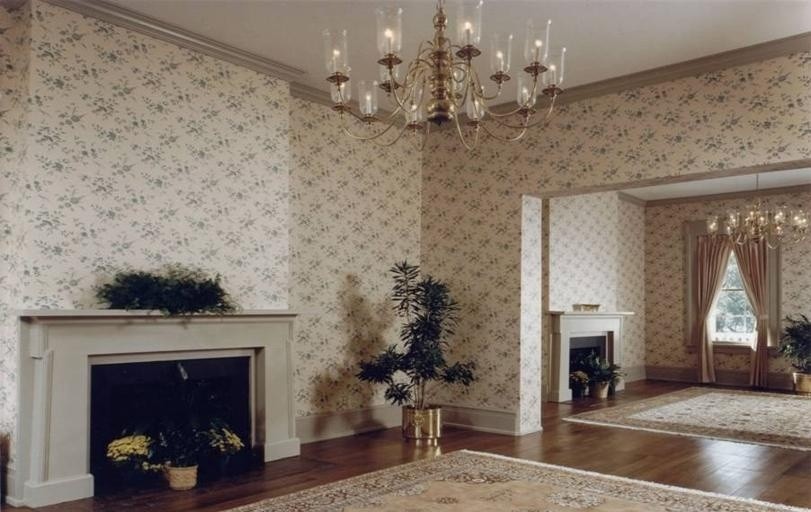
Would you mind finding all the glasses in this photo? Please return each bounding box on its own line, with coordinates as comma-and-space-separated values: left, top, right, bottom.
321, 2, 568, 153
705, 174, 811, 251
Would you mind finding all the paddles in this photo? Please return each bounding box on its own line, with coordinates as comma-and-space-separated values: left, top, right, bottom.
224, 448, 809, 512
562, 384, 811, 453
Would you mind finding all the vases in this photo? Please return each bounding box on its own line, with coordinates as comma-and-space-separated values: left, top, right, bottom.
107, 434, 164, 471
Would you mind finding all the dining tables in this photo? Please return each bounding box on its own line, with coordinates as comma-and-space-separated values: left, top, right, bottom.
156, 415, 247, 492
774, 313, 811, 393
569, 349, 627, 400
355, 248, 481, 442
